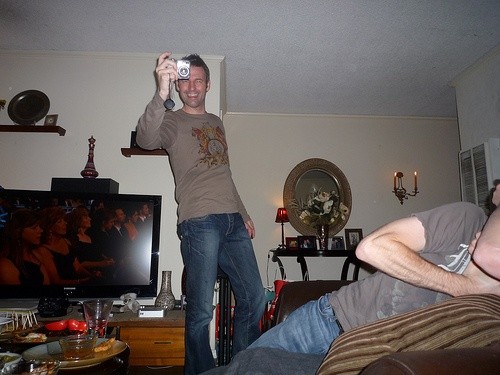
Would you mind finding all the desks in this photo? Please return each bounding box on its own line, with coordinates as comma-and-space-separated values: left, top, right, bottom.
0, 306, 37, 328
270, 247, 359, 281
23, 338, 130, 375
0, 318, 14, 331
0, 330, 84, 353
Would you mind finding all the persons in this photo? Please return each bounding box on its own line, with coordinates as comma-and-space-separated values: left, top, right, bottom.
301, 239, 313, 248
137, 51, 265, 375
0, 199, 149, 285
201, 183, 500, 375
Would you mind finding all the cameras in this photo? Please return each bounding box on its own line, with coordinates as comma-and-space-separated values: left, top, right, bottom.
167, 58, 191, 80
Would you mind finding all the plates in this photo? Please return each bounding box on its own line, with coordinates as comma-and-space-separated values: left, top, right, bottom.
8, 90, 50, 125
22, 338, 127, 369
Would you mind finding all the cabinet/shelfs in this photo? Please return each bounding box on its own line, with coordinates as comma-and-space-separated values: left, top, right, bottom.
38, 308, 186, 366
0, 125, 169, 158
458, 141, 493, 207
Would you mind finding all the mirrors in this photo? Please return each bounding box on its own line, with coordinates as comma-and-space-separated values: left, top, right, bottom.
283, 158, 352, 237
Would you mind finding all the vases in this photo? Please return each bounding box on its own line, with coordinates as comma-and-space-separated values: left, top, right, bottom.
316, 225, 330, 250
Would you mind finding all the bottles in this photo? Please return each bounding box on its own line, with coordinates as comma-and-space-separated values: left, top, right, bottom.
155, 271, 176, 311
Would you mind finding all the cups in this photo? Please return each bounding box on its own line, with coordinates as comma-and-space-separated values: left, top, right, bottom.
83, 299, 113, 338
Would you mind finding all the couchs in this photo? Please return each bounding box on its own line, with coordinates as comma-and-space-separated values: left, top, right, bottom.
270, 279, 500, 375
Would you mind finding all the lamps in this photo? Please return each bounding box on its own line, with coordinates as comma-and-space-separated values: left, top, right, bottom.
275, 208, 289, 248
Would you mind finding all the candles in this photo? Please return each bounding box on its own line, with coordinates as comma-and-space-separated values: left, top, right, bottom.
394, 176, 396, 190
414, 171, 418, 188
398, 177, 401, 188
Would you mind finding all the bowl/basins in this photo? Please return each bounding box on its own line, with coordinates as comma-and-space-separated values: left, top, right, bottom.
0, 353, 22, 375
60, 333, 98, 360
0, 359, 61, 375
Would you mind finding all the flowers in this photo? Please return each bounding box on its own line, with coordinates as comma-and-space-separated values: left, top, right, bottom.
288, 188, 349, 224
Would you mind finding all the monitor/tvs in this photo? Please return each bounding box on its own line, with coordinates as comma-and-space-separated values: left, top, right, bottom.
0, 187, 161, 314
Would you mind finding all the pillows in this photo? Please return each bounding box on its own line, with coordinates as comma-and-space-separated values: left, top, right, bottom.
317, 293, 500, 375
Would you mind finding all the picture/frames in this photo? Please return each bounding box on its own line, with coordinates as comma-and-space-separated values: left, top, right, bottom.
330, 229, 363, 250
286, 236, 316, 248
